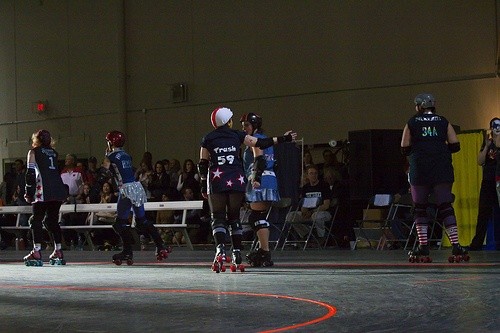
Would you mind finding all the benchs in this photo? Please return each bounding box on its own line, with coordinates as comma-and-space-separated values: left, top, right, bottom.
0, 201, 203, 250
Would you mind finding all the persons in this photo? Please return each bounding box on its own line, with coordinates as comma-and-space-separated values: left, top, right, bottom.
24, 129, 66, 266
401, 92, 470, 263
238, 113, 281, 267
285, 148, 350, 245
397, 152, 422, 207
93, 131, 173, 266
197, 107, 297, 272
0, 152, 217, 251
469, 118, 500, 249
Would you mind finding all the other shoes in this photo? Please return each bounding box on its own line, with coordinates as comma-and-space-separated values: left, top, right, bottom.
465, 244, 481, 251
308, 240, 316, 248
103, 242, 111, 251
311, 238, 325, 248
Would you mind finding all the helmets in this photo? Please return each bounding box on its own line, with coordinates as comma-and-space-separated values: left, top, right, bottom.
239, 112, 262, 129
415, 93, 435, 108
105, 131, 125, 147
211, 107, 234, 129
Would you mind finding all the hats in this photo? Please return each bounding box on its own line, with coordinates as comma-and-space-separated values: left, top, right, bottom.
35, 129, 51, 146
88, 156, 97, 163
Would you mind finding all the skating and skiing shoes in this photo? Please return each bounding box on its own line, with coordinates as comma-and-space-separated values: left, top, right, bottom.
49, 249, 66, 265
230, 251, 244, 272
155, 241, 172, 261
23, 249, 43, 266
211, 243, 230, 272
408, 244, 432, 263
112, 249, 133, 265
246, 247, 273, 267
448, 242, 470, 263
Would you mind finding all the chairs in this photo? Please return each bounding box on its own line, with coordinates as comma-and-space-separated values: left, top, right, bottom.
240, 191, 445, 250
